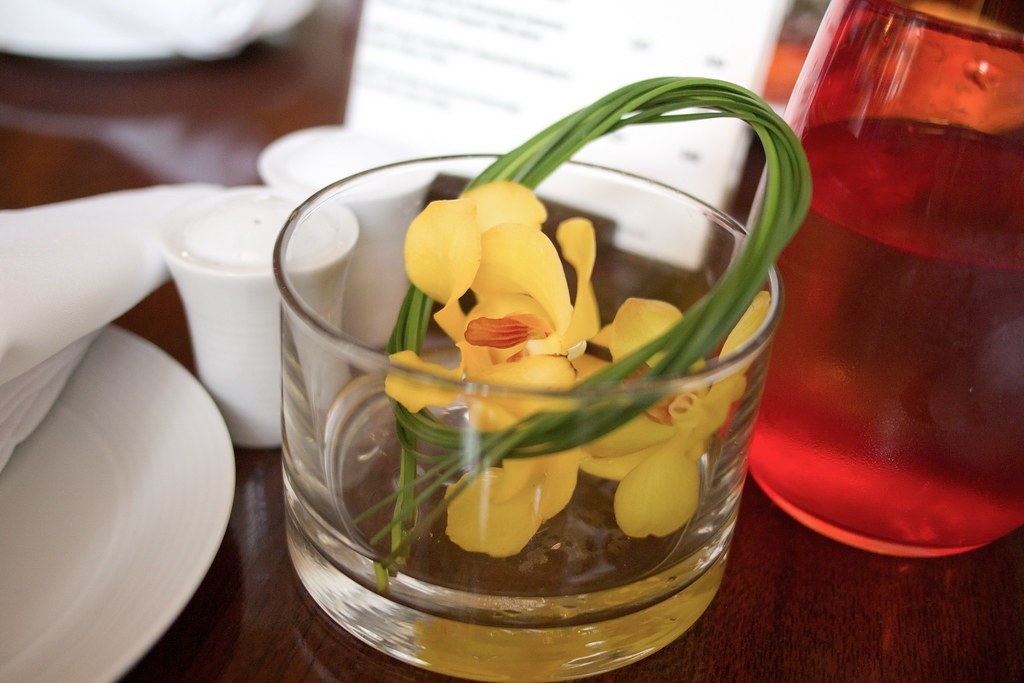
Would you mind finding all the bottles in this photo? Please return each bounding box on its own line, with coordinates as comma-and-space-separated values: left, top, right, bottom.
745, 0, 1024, 560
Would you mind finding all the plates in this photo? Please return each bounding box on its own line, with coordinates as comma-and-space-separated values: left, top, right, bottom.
0, 323, 235, 683
0, 0, 320, 70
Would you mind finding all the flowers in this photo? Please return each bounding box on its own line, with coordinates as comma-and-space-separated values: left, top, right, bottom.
384, 168, 778, 563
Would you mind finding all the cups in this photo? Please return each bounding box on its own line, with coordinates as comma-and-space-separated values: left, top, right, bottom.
158, 188, 361, 450
257, 126, 444, 351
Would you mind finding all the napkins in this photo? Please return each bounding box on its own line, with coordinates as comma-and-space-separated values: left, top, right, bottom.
0, 180, 228, 476
